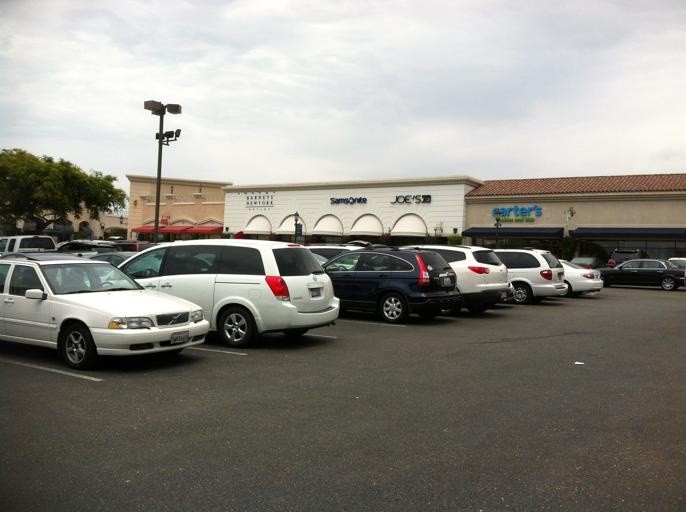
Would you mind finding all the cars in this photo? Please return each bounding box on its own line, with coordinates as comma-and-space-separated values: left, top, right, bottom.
300, 241, 364, 271
558, 259, 604, 296
571, 256, 605, 268
0, 252, 211, 370
0, 230, 213, 284
594, 258, 686, 292
667, 257, 686, 285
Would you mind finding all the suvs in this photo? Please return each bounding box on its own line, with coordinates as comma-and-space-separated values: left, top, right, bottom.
607, 248, 651, 268
397, 244, 515, 315
321, 244, 464, 323
490, 247, 569, 305
86, 238, 341, 347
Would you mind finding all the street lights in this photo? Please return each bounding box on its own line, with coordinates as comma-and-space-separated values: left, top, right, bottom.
141, 98, 182, 246
